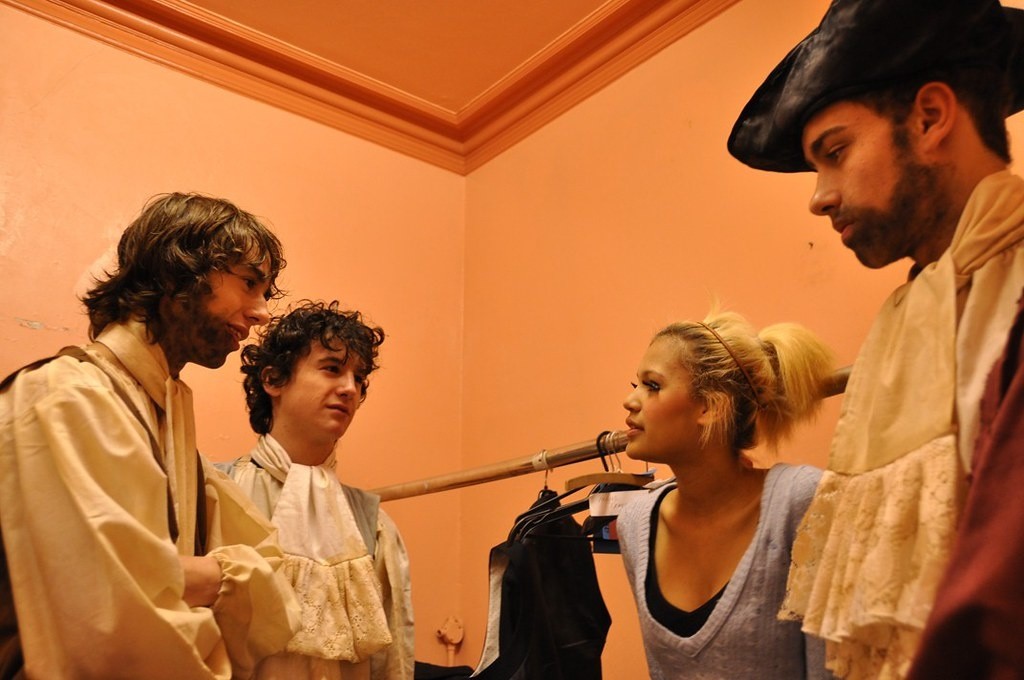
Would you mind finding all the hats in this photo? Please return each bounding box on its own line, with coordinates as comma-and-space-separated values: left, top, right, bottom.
726, 1, 1024, 173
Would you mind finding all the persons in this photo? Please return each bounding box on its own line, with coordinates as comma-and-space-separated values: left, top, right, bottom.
0, 194, 286, 679
616, 314, 842, 680
204, 299, 414, 680
728, 0, 1024, 680
